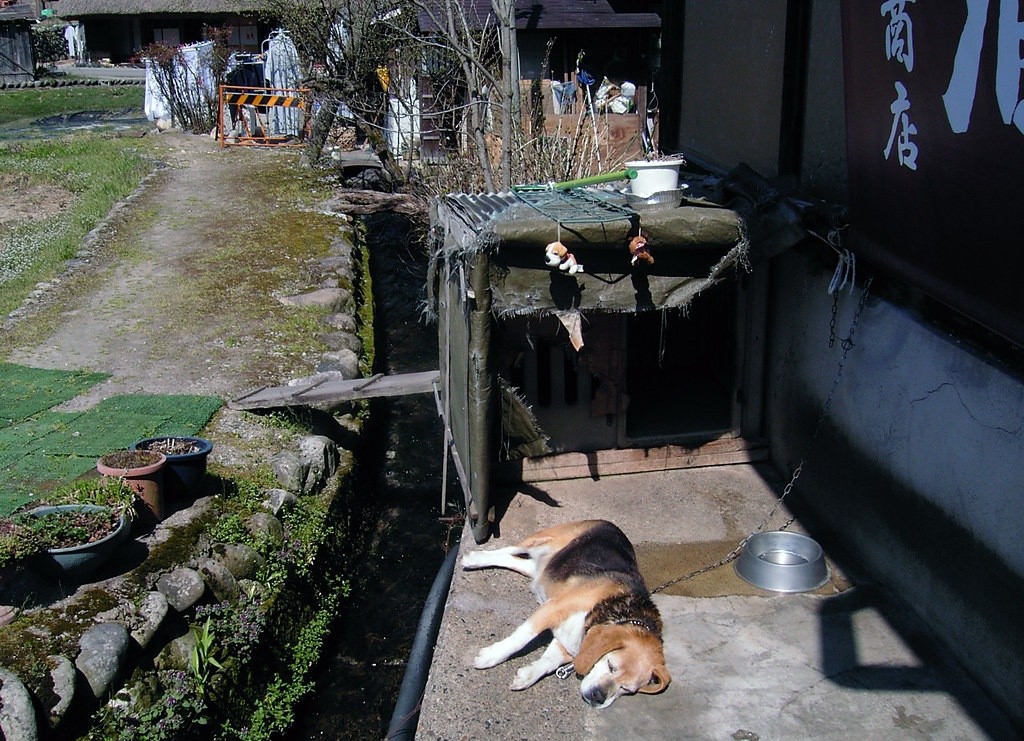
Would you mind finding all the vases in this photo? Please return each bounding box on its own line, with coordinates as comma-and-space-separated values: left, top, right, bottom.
130, 437, 213, 493
97, 450, 166, 525
25, 504, 124, 576
624, 160, 682, 197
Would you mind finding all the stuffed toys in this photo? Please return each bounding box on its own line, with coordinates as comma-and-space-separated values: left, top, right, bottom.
544, 241, 579, 274
629, 236, 654, 267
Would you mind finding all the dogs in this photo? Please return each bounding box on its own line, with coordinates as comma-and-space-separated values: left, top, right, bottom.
460, 519, 672, 710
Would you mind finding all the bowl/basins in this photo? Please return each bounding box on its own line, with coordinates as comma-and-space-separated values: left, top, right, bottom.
619, 183, 689, 212
732, 530, 833, 592
